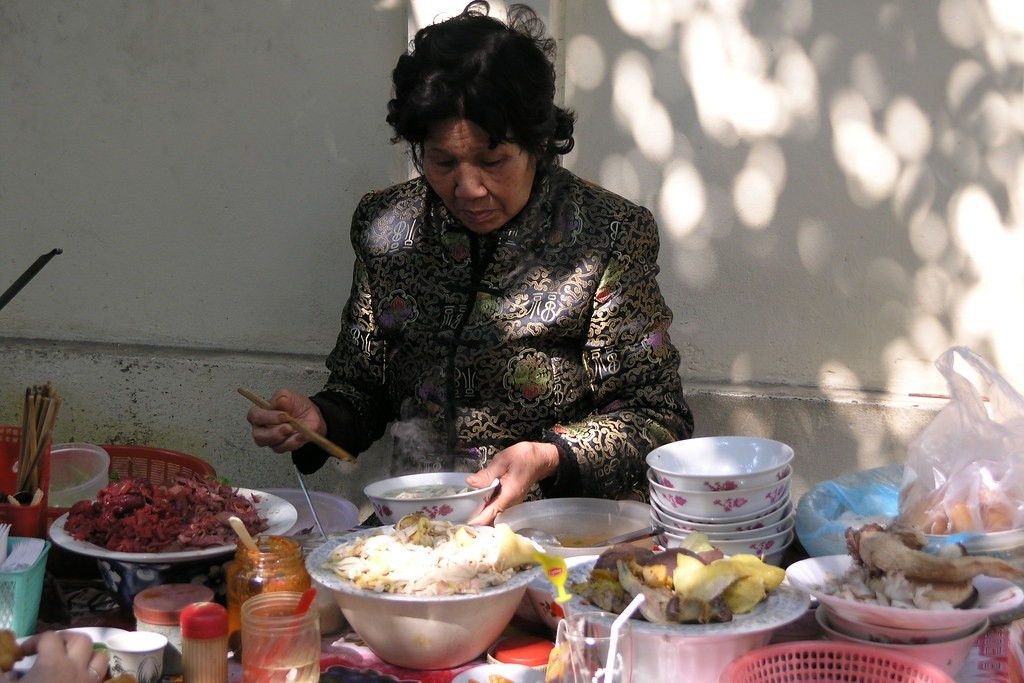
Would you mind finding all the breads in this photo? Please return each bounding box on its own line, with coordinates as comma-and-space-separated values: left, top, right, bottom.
903, 493, 1015, 534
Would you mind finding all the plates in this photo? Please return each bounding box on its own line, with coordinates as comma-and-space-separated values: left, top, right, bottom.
305, 523, 546, 601
550, 558, 811, 636
786, 554, 1024, 630
50, 487, 298, 563
451, 663, 545, 683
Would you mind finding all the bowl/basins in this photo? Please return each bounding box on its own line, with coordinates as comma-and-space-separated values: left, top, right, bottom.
594, 625, 772, 683
527, 587, 564, 629
815, 605, 989, 679
333, 582, 529, 669
363, 472, 500, 525
96, 552, 234, 614
13, 627, 128, 680
645, 436, 795, 566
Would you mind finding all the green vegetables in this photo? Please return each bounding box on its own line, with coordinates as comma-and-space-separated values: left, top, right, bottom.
206, 473, 230, 486
108, 471, 121, 483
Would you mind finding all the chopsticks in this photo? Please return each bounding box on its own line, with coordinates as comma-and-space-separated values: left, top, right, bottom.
16, 380, 62, 495
238, 386, 352, 461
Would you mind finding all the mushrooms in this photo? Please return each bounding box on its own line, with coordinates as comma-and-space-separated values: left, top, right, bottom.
831, 522, 1024, 611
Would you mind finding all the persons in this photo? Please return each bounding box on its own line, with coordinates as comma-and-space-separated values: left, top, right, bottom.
0, 629, 109, 683
247, 0, 695, 528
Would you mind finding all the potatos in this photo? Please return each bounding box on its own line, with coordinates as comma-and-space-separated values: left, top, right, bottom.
590, 546, 726, 590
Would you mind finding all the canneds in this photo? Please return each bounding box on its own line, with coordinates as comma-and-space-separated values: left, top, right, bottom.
224, 534, 313, 662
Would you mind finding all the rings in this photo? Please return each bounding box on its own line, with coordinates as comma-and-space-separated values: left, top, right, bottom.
88, 666, 103, 682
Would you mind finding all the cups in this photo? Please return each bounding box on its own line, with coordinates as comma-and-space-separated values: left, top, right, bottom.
47, 442, 110, 506
106, 631, 168, 683
241, 591, 321, 683
255, 488, 360, 637
557, 612, 633, 683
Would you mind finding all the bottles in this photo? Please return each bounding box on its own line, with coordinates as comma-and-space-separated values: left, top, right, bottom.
225, 535, 311, 664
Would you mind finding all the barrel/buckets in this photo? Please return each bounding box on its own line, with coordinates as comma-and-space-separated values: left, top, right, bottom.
922, 527, 1024, 586
494, 497, 655, 558
